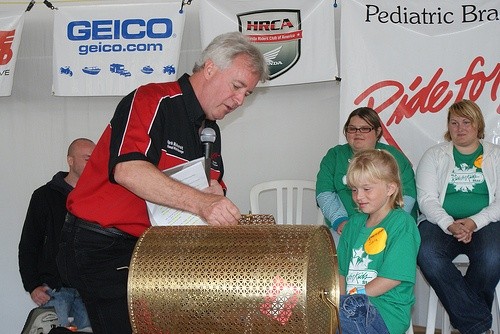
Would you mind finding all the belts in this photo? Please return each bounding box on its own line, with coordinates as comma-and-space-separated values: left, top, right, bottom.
64, 212, 138, 242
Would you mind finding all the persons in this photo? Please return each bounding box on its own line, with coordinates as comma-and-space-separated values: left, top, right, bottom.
64, 31, 268, 334
315, 107, 417, 256
338, 148, 421, 334
18, 137, 96, 332
416, 100, 500, 334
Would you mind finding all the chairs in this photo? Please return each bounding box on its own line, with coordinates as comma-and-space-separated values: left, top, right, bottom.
250, 179, 325, 226
425, 253, 500, 334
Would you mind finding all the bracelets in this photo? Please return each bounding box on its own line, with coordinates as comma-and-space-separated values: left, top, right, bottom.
349, 286, 366, 295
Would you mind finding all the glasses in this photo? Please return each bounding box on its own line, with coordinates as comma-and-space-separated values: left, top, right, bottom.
345, 127, 374, 133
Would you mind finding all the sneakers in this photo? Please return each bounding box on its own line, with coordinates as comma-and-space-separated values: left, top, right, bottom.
21, 306, 60, 334
